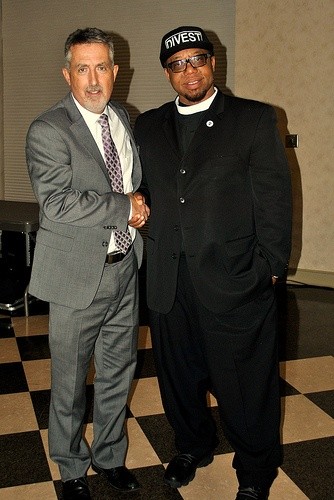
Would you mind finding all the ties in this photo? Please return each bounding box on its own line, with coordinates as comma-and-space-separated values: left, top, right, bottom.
97, 114, 133, 255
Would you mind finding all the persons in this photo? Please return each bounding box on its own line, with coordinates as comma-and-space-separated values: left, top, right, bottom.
127, 26, 293, 500
26, 28, 150, 500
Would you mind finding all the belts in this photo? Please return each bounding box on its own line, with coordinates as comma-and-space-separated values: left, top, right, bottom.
106, 245, 132, 264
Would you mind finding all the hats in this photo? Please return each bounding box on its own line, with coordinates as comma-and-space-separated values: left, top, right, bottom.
159, 26, 214, 68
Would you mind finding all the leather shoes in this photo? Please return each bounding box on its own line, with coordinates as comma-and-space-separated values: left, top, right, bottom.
162, 453, 214, 488
235, 485, 270, 500
91, 465, 141, 493
64, 477, 91, 500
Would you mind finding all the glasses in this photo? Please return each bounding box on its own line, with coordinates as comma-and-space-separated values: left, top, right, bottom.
165, 53, 213, 73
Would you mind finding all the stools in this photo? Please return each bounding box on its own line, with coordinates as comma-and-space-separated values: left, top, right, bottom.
0, 261, 42, 317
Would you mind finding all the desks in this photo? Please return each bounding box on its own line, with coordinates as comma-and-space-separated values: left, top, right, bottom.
0, 200, 40, 267
